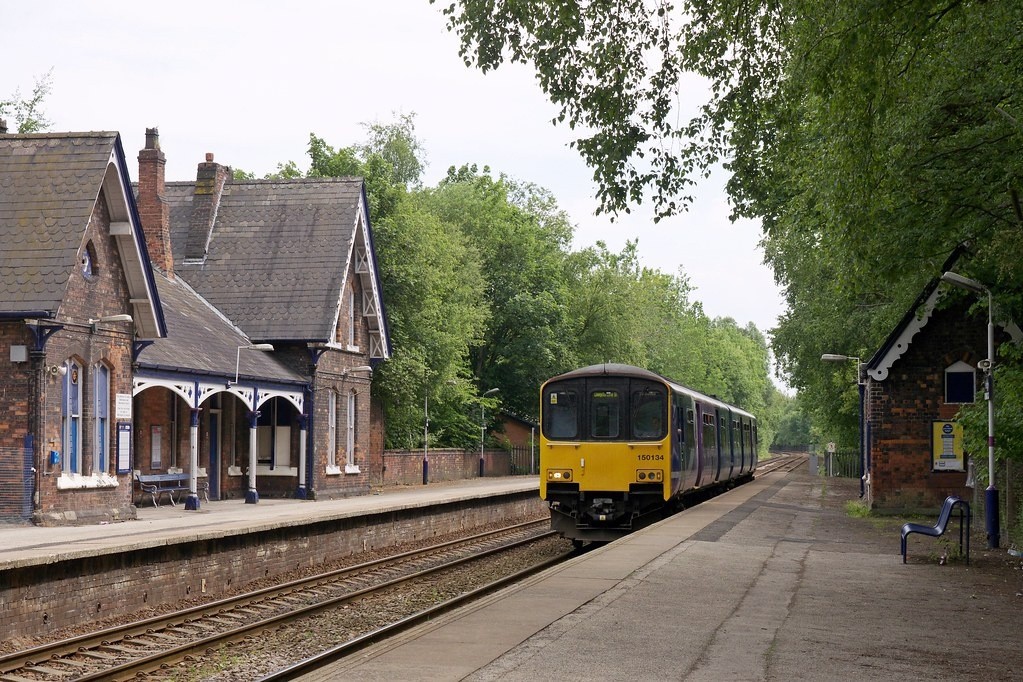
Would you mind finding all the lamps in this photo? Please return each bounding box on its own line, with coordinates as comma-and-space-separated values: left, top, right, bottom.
821, 354, 869, 385
344, 366, 373, 375
89, 315, 133, 324
226, 343, 275, 386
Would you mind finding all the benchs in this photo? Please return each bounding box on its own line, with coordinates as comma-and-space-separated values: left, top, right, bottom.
136, 473, 209, 509
901, 495, 970, 565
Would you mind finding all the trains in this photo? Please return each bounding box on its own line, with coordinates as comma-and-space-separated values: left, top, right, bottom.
539, 356, 758, 549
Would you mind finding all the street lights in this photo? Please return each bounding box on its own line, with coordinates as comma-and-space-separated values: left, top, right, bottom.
423, 378, 457, 486
942, 272, 999, 548
820, 354, 864, 498
480, 388, 500, 477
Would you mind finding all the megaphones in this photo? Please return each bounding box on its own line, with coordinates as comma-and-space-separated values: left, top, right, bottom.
977, 359, 992, 372
50, 366, 58, 375
59, 366, 66, 376
